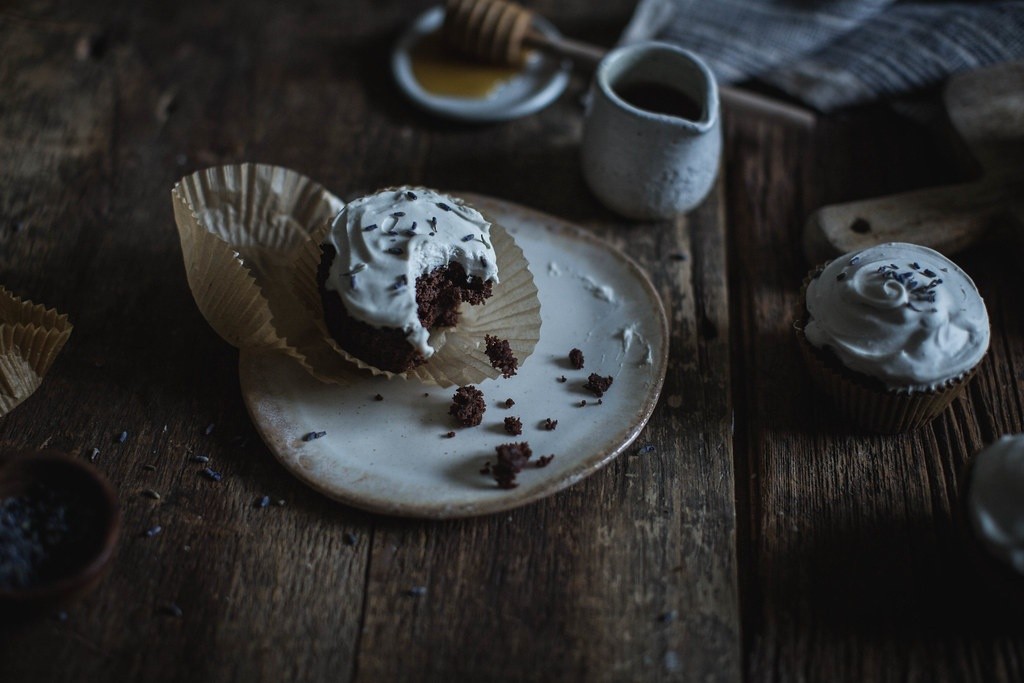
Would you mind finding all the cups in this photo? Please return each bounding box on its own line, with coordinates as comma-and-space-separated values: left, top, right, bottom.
582, 41, 721, 220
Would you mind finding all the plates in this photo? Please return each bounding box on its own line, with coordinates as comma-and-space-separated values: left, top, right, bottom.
236, 191, 668, 520
392, 2, 567, 124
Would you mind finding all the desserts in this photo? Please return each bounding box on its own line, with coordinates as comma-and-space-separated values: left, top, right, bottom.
293, 184, 542, 388
793, 239, 991, 433
